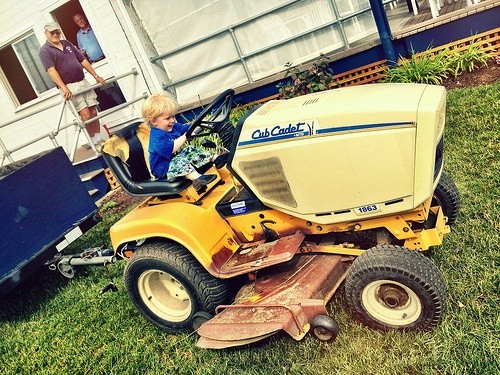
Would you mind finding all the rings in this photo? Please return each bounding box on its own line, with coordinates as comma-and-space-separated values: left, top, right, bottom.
66, 92, 69, 95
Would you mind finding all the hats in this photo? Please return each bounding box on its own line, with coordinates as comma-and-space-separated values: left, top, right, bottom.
44, 22, 61, 33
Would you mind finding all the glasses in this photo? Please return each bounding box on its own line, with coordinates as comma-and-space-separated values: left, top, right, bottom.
47, 31, 60, 35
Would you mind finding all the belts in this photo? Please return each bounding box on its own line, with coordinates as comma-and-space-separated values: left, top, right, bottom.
90, 55, 105, 64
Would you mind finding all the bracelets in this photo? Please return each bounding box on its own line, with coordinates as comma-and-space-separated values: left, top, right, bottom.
94, 74, 99, 79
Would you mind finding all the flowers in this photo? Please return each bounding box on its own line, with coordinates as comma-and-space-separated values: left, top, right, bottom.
294, 66, 317, 90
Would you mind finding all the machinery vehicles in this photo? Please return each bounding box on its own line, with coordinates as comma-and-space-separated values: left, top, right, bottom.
100, 82, 461, 348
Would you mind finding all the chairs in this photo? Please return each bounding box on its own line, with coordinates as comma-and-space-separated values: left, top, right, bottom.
101, 121, 214, 197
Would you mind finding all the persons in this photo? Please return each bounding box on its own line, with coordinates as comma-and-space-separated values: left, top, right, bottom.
142, 92, 232, 193
72, 12, 126, 106
39, 22, 110, 153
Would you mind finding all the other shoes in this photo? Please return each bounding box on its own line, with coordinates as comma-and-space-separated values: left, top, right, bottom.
213, 153, 231, 169
95, 138, 106, 153
193, 174, 217, 189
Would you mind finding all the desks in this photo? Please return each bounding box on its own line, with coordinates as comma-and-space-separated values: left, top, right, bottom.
282, 0, 398, 58
